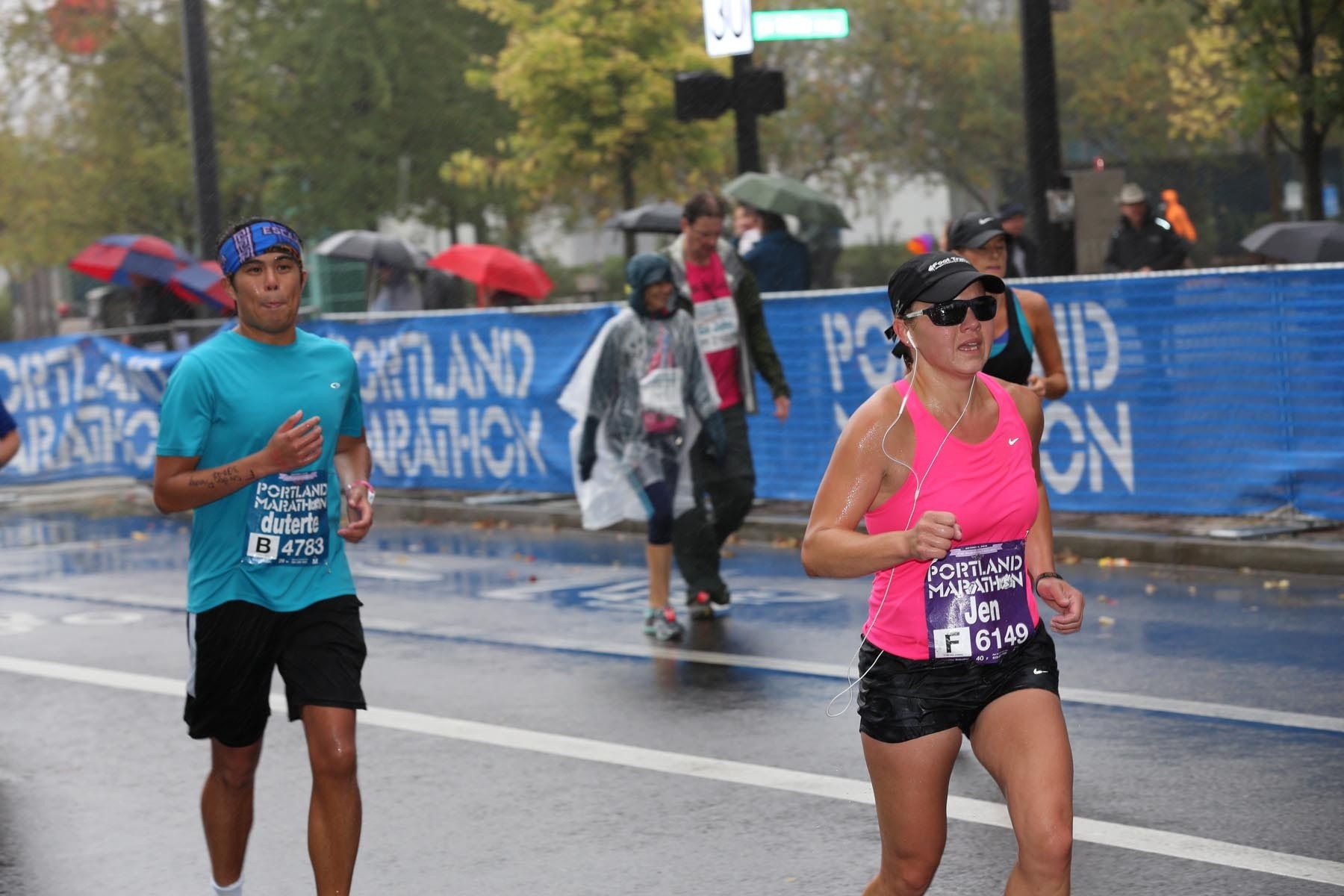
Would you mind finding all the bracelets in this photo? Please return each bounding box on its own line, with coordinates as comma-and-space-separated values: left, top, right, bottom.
344, 480, 376, 505
1035, 572, 1063, 597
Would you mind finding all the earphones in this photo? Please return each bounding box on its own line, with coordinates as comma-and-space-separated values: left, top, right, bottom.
907, 332, 916, 349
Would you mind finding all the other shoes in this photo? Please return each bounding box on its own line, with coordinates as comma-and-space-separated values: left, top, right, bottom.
641, 607, 686, 642
685, 589, 716, 620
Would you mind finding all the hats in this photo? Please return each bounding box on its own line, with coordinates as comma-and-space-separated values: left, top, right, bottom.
887, 250, 1007, 360
948, 212, 1013, 251
1118, 180, 1149, 205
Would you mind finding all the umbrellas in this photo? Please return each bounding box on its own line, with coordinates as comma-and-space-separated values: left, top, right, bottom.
603, 202, 686, 234
718, 171, 851, 229
167, 260, 236, 317
67, 232, 197, 290
310, 228, 421, 274
1238, 219, 1344, 262
428, 243, 556, 307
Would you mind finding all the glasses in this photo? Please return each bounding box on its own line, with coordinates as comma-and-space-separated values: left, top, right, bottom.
884, 296, 999, 338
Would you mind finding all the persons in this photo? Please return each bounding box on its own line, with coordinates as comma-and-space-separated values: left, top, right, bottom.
490, 291, 531, 307
154, 218, 377, 896
943, 211, 1068, 400
800, 251, 1086, 896
368, 264, 465, 313
657, 189, 790, 617
727, 201, 815, 292
997, 203, 1039, 279
554, 250, 728, 641
1104, 181, 1200, 272
102, 280, 215, 349
0, 399, 21, 469
